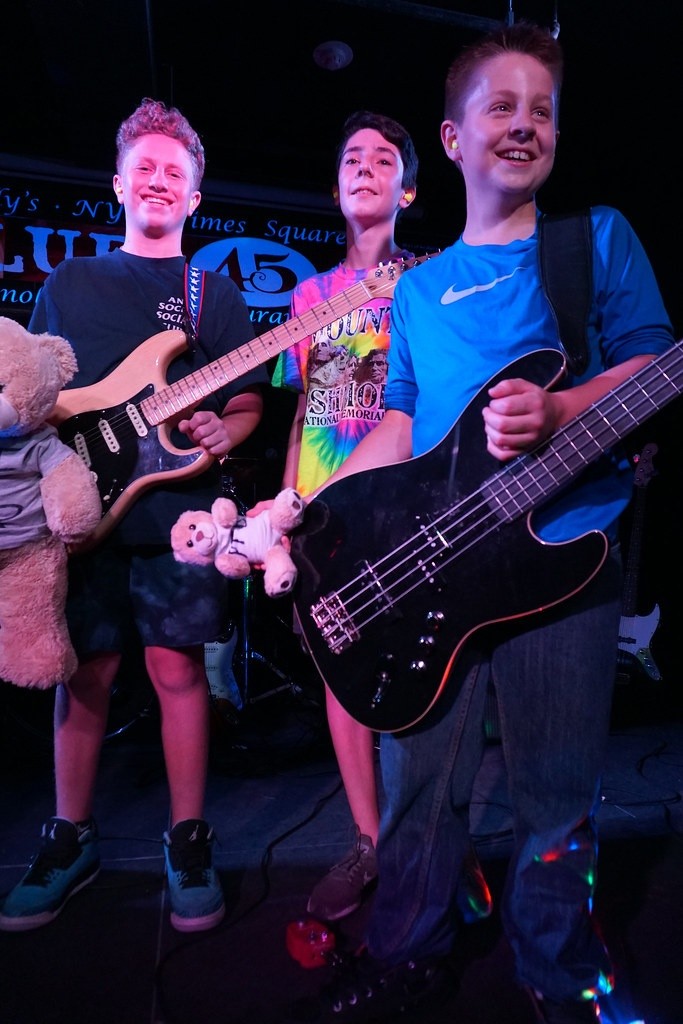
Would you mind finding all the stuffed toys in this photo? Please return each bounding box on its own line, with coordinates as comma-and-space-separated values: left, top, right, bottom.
0, 316, 102, 688
172, 487, 305, 597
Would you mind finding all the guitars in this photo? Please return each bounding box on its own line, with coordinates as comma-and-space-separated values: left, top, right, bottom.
279, 334, 683, 736
52, 246, 446, 546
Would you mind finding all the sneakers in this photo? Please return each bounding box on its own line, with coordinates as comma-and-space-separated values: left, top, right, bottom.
163, 818, 227, 934
305, 832, 380, 921
284, 960, 462, 1024
1, 816, 103, 931
524, 976, 600, 1024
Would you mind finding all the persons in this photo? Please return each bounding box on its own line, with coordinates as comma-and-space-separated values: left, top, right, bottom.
272, 22, 673, 1024
0, 98, 268, 931
